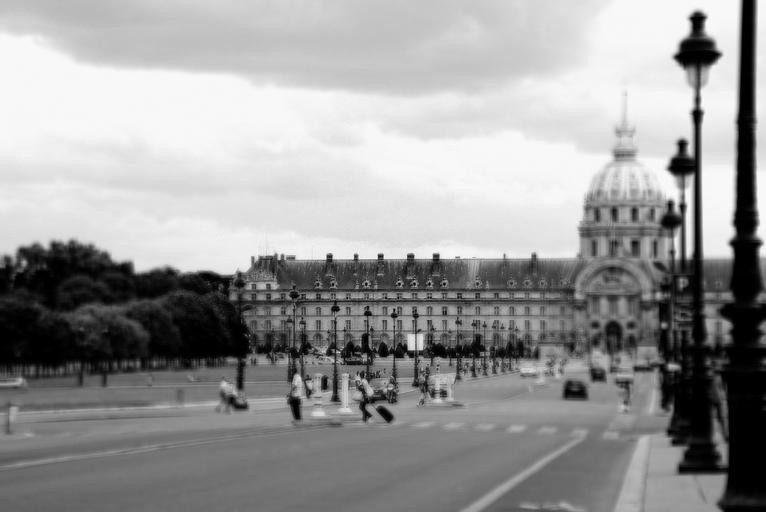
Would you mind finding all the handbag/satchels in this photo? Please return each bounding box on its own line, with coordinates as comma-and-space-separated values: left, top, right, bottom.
353, 391, 365, 402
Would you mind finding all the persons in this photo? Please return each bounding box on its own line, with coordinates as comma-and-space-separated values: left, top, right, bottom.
286, 370, 303, 425
352, 356, 503, 408
304, 374, 318, 397
216, 376, 234, 415
357, 372, 376, 422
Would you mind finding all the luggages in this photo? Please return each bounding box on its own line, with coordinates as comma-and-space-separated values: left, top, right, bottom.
369, 401, 393, 422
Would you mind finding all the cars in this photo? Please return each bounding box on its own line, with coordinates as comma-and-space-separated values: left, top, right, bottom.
521, 365, 539, 377
592, 367, 607, 383
0, 376, 28, 389
634, 359, 655, 372
562, 379, 588, 400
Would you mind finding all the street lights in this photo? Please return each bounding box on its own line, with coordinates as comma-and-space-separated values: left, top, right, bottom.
286, 283, 518, 403
652, 9, 729, 473
234, 273, 247, 390
